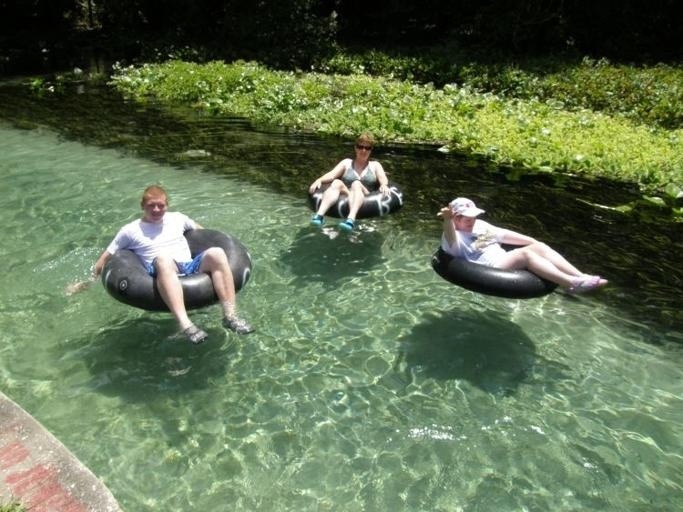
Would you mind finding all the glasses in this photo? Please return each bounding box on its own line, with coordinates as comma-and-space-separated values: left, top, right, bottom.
356, 143, 373, 151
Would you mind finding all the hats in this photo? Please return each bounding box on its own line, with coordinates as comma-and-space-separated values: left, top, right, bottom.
449, 197, 485, 219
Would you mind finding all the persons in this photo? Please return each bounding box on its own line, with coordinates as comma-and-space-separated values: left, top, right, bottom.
306, 131, 388, 232
64, 185, 257, 345
435, 198, 609, 296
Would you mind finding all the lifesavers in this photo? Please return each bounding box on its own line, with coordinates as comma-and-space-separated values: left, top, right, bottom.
431, 246, 558, 298
102, 229, 252, 311
306, 180, 404, 219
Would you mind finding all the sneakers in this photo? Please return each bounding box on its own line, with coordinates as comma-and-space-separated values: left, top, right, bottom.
309, 214, 325, 227
563, 274, 608, 294
338, 218, 354, 233
186, 327, 209, 344
222, 316, 255, 335
